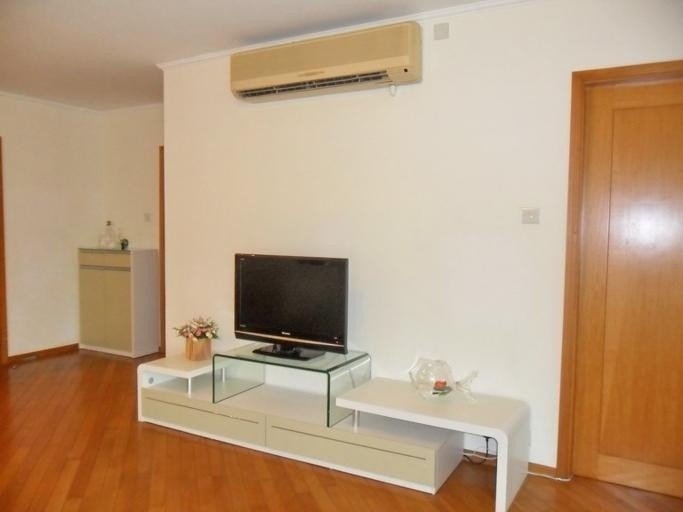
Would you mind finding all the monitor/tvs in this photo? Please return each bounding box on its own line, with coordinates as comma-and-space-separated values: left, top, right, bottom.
235, 253, 349, 360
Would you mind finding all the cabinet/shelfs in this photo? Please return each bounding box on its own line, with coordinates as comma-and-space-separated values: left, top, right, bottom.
76, 244, 161, 359
137, 343, 533, 512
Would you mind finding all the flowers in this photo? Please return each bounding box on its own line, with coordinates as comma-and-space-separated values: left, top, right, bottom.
169, 313, 221, 343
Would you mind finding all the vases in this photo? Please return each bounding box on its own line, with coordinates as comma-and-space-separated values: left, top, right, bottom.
182, 336, 212, 361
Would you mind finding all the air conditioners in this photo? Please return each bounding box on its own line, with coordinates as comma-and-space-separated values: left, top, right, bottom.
226, 20, 423, 104
